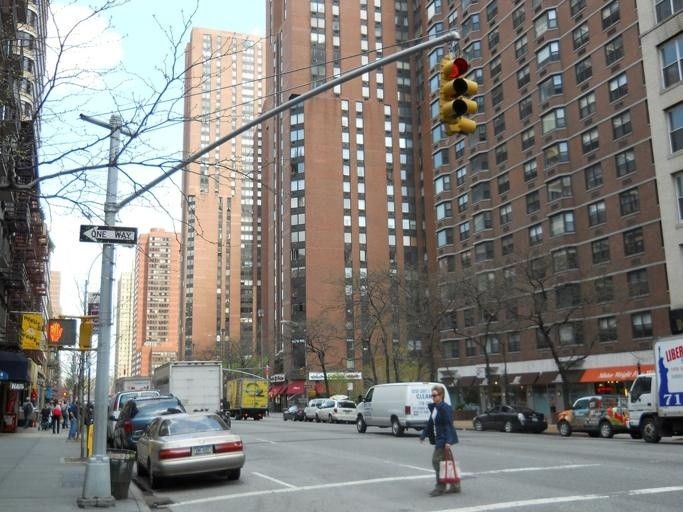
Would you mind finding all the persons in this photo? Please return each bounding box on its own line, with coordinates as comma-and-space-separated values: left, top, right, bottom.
61, 400, 69, 429
21, 397, 35, 429
67, 400, 80, 440
51, 403, 61, 433
52, 399, 61, 429
419, 385, 461, 497
39, 403, 50, 430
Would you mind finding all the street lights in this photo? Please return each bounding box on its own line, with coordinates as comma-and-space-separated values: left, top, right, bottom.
214, 328, 229, 358
256, 306, 265, 378
280, 318, 309, 404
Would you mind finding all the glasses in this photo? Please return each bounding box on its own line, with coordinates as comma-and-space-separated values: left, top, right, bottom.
430, 394, 439, 397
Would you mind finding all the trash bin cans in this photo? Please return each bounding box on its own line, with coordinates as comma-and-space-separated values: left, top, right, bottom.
4, 412, 17, 433
106, 448, 137, 500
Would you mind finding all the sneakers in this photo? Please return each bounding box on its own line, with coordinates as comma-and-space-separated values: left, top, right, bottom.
431, 489, 443, 496
446, 487, 461, 494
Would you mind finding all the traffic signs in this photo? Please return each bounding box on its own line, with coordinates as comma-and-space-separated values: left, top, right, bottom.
77, 223, 138, 245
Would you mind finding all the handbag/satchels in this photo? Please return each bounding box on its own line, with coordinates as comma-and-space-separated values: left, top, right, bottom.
25, 403, 33, 414
53, 408, 60, 416
438, 449, 462, 482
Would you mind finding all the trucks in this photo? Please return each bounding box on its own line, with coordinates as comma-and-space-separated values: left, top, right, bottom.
354, 381, 453, 437
113, 375, 153, 393
221, 377, 270, 421
148, 358, 225, 419
624, 332, 682, 444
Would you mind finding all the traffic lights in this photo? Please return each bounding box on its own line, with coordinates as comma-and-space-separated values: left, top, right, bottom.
443, 76, 483, 137
435, 52, 470, 127
44, 318, 78, 349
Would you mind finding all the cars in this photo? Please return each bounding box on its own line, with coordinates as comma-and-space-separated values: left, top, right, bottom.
281, 396, 358, 424
470, 403, 547, 434
77, 390, 246, 488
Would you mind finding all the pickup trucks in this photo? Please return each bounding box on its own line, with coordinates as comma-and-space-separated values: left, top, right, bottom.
550, 394, 630, 438
